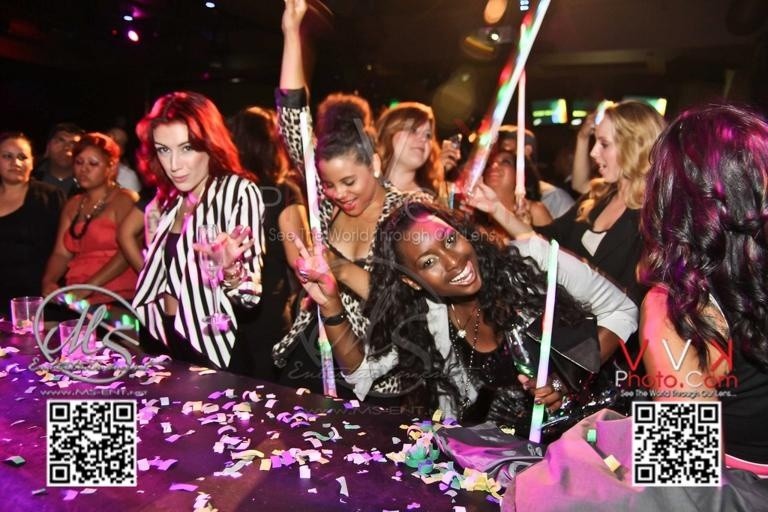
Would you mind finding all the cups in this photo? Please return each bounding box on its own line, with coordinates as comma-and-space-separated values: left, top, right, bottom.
56, 319, 98, 363
10, 295, 47, 335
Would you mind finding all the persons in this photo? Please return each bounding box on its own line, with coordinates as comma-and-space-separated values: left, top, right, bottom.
2, 0, 767, 469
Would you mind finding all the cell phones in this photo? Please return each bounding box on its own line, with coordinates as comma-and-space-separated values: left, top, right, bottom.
595, 99, 612, 126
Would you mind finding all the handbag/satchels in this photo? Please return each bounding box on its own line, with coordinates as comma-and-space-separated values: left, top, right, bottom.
525, 305, 600, 392
435, 420, 545, 494
500, 409, 767, 512
